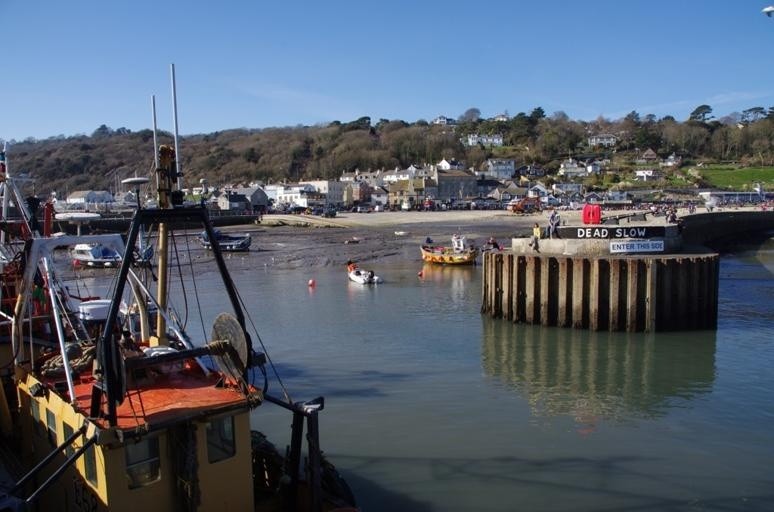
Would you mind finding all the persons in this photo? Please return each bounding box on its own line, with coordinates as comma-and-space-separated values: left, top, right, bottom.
487, 236, 499, 252
529, 222, 540, 246
347, 258, 358, 272
600, 198, 774, 222
544, 209, 560, 239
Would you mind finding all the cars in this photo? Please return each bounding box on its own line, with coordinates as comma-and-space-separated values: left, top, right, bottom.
450, 199, 510, 210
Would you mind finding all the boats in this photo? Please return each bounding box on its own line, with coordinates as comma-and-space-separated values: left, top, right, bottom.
198, 228, 251, 251
67, 243, 153, 267
349, 268, 382, 284
419, 245, 478, 264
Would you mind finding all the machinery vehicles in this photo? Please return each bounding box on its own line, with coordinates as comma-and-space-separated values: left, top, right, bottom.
510, 196, 543, 216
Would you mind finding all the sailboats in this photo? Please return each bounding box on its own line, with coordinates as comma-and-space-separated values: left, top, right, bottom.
0, 61, 356, 512
